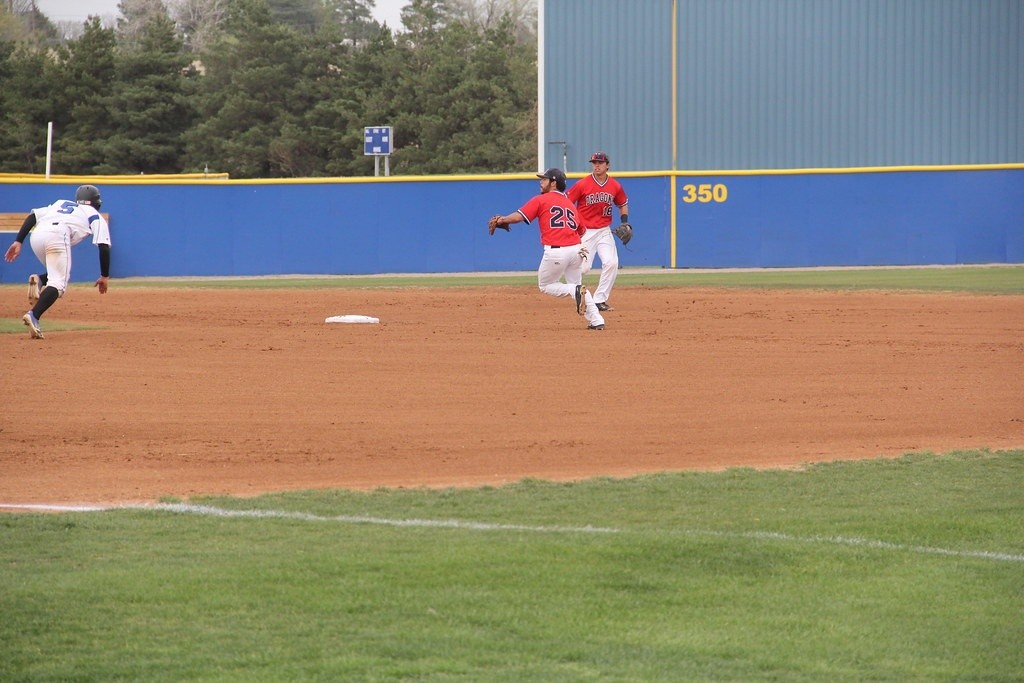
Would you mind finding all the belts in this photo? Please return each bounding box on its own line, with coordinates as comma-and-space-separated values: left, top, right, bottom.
551, 246, 560, 248
53, 222, 58, 225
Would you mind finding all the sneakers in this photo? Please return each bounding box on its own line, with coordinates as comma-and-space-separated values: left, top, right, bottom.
588, 325, 605, 330
596, 303, 614, 310
23, 310, 43, 339
27, 274, 42, 305
576, 285, 587, 315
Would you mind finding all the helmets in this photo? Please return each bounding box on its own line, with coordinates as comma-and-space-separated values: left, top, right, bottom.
75, 185, 102, 206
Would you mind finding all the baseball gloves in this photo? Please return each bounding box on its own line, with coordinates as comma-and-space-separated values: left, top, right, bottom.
610, 221, 634, 245
488, 214, 512, 236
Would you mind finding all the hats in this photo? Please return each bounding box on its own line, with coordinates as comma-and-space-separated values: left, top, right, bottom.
589, 152, 609, 163
537, 167, 566, 183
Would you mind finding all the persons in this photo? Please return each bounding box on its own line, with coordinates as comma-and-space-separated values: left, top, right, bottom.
4, 185, 111, 339
564, 152, 632, 313
489, 168, 604, 331
